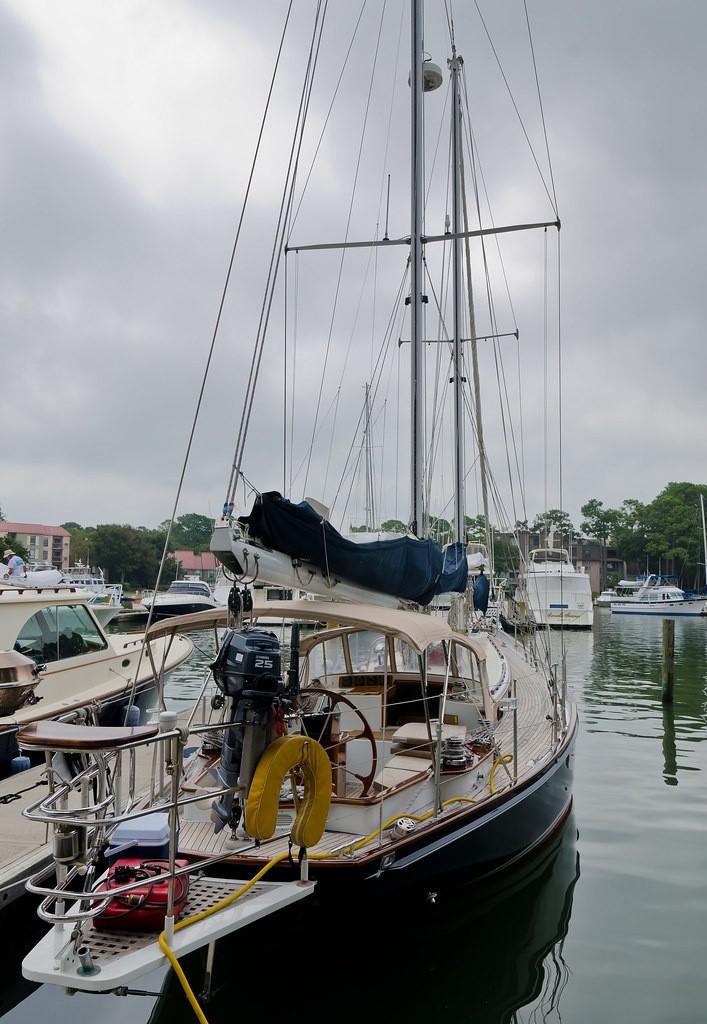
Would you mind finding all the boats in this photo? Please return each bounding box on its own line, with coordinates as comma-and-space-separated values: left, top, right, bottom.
0, 563, 329, 918
160, 796, 580, 1023
498, 523, 594, 630
594, 575, 650, 608
610, 574, 707, 616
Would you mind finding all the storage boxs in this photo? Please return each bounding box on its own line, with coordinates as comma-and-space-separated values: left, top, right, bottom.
105, 812, 179, 870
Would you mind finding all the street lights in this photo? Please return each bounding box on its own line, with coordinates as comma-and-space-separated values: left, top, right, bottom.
85, 538, 90, 565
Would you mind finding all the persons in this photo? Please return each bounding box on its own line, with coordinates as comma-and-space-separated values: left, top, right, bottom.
3, 550, 27, 579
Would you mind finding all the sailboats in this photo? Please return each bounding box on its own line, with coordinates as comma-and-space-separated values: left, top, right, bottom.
16, 1, 581, 1024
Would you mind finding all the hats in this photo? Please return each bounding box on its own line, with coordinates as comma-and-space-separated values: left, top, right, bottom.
3, 549, 16, 558
